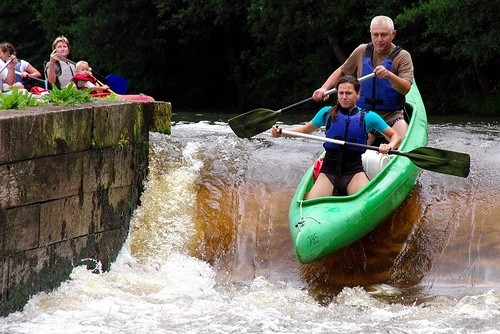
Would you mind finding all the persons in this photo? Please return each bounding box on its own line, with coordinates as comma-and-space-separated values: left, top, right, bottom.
272, 75, 404, 200
312, 16, 413, 150
0, 35, 110, 98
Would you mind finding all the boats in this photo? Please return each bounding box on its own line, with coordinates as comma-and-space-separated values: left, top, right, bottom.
288, 75, 429, 264
31, 86, 155, 102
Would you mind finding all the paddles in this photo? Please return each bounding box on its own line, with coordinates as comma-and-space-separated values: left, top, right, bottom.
57, 55, 128, 95
226, 71, 379, 139
276, 128, 471, 180
